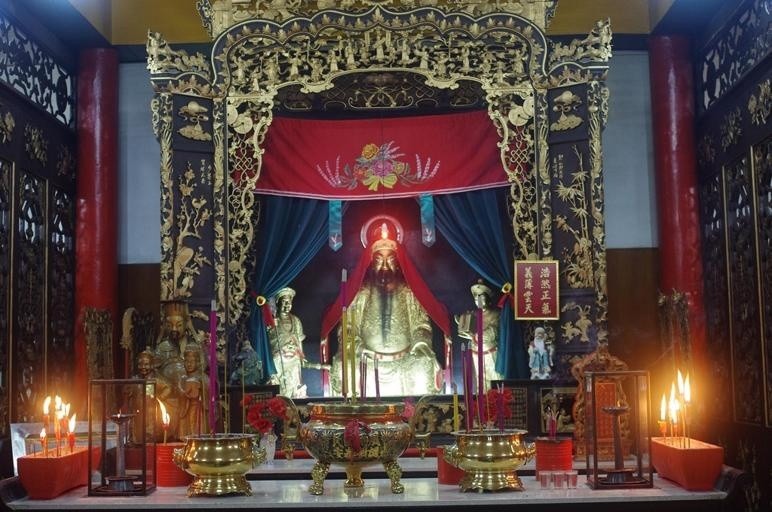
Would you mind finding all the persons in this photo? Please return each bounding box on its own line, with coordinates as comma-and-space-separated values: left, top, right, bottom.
529, 328, 554, 380
122, 346, 173, 443
542, 410, 572, 432
153, 303, 211, 441
330, 227, 441, 396
453, 279, 506, 394
267, 287, 308, 399
179, 344, 220, 440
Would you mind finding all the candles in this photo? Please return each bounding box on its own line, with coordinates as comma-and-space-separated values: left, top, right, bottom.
157, 398, 171, 431
40, 395, 77, 453
657, 366, 691, 431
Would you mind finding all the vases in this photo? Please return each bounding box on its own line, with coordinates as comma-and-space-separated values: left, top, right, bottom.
259, 431, 276, 465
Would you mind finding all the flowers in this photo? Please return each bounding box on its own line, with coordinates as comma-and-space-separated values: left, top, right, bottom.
471, 387, 515, 425
239, 395, 289, 435
